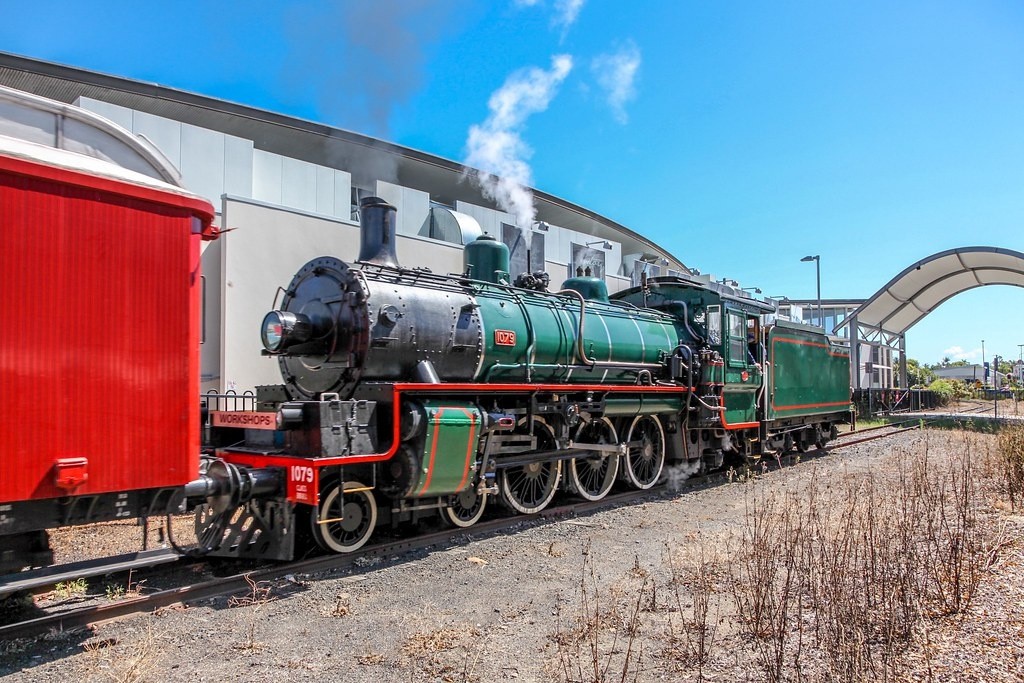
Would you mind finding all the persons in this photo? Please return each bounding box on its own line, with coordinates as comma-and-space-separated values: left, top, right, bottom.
747, 333, 762, 370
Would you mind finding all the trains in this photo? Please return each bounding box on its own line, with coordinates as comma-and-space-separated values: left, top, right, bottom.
0, 84, 857, 626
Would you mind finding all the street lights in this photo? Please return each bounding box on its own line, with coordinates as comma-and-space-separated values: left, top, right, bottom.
799, 255, 821, 325
